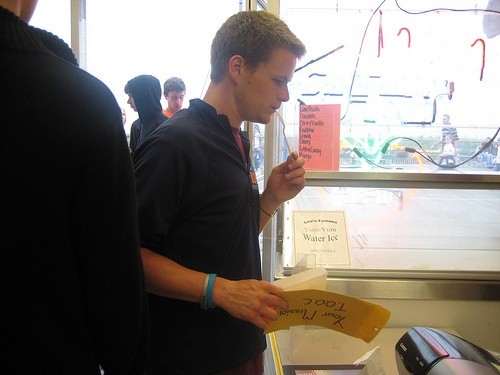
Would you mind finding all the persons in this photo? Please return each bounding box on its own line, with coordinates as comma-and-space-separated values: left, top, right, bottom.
125, 74, 171, 151
472, 136, 500, 171
440, 115, 460, 168
162, 77, 186, 120
1, 1, 146, 375
129, 10, 306, 375
439, 134, 457, 168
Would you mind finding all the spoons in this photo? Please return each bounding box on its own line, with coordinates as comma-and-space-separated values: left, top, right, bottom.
274, 109, 294, 162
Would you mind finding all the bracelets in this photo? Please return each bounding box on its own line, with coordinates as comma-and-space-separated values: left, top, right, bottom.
259, 194, 277, 216
206, 273, 217, 308
200, 273, 211, 310
